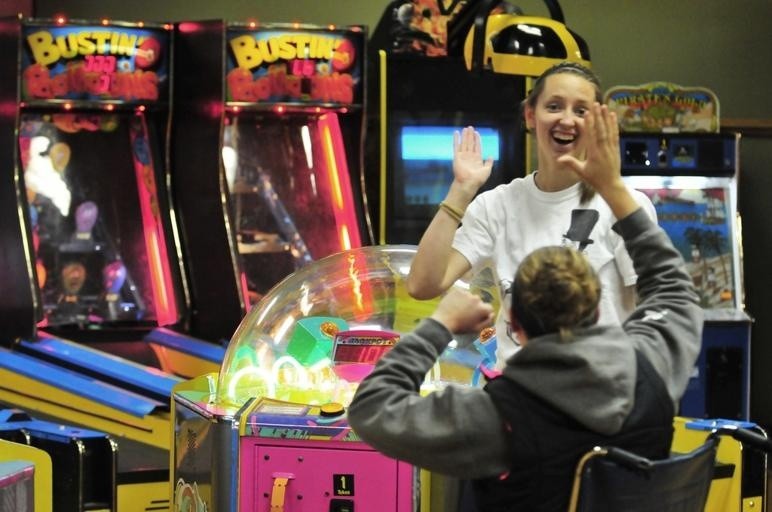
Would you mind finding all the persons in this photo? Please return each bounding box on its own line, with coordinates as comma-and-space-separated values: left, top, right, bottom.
322, 98, 705, 510
402, 58, 657, 362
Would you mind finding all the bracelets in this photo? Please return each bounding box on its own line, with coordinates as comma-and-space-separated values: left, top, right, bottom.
437, 203, 463, 223
439, 200, 466, 218
